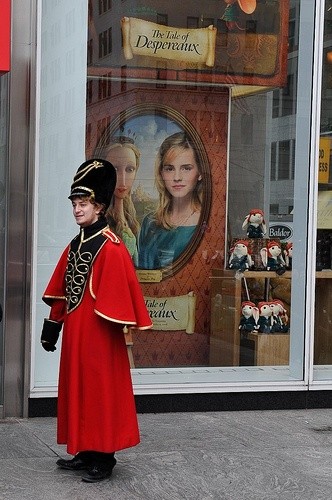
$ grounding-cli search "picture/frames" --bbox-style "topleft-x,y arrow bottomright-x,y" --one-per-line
94,102 -> 212,283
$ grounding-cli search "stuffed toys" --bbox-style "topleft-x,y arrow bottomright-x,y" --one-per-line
239,299 -> 289,336
228,208 -> 292,275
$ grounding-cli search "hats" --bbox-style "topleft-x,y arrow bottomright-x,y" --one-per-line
68,158 -> 116,211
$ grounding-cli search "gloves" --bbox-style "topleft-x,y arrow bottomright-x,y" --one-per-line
40,318 -> 62,352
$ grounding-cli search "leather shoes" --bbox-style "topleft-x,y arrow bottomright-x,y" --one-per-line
56,452 -> 96,469
82,466 -> 113,483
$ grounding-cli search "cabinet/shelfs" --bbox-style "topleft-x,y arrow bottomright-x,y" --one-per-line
208,268 -> 332,367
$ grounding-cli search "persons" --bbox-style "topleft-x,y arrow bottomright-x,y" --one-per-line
39,158 -> 155,484
132,131 -> 207,270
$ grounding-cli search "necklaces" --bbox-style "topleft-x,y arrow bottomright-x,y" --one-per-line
161,202 -> 198,229
